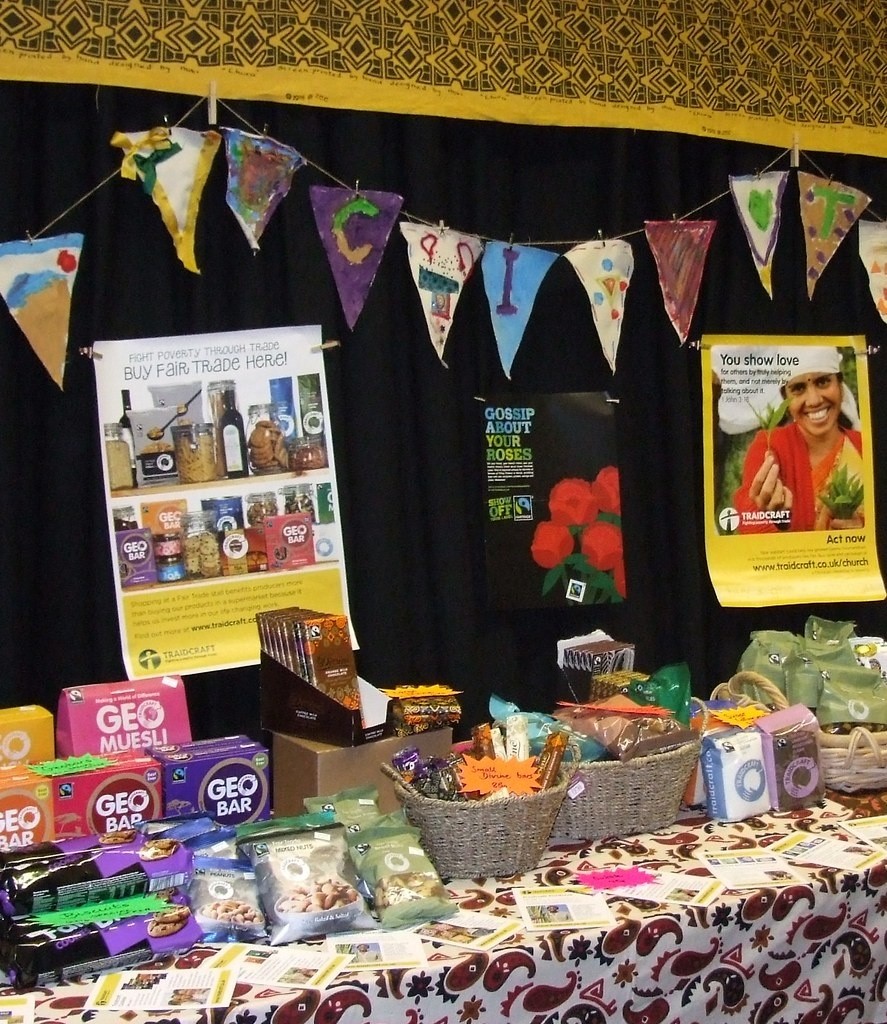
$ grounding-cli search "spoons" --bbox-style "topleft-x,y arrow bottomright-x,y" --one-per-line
147,414 -> 177,440
178,390 -> 201,416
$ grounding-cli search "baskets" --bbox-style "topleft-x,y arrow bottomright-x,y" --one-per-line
381,740 -> 581,880
543,696 -> 708,848
718,670 -> 887,792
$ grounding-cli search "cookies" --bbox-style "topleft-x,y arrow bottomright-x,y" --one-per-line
182,533 -> 220,578
248,420 -> 289,469
98,829 -> 191,938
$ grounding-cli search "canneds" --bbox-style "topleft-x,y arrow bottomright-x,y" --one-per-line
152,532 -> 188,584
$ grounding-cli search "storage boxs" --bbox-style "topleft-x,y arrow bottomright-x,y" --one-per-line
271,727 -> 454,815
0,749 -> 163,850
260,650 -> 393,748
144,734 -> 261,817
0,705 -> 55,767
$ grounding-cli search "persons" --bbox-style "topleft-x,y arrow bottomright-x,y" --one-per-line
545,905 -> 571,922
168,988 -> 211,1006
351,944 -> 379,963
430,921 -> 478,938
732,347 -> 865,535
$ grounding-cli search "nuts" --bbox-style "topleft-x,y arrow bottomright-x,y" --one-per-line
200,878 -> 360,925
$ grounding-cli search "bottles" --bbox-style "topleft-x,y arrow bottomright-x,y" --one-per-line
105,423 -> 133,491
277,482 -> 315,524
113,506 -> 138,533
220,388 -> 248,478
181,510 -> 221,581
247,404 -> 289,477
288,436 -> 327,471
207,379 -> 236,478
247,491 -> 278,531
120,389 -> 138,487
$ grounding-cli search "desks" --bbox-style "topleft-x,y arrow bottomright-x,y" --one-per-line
0,786 -> 887,1024
